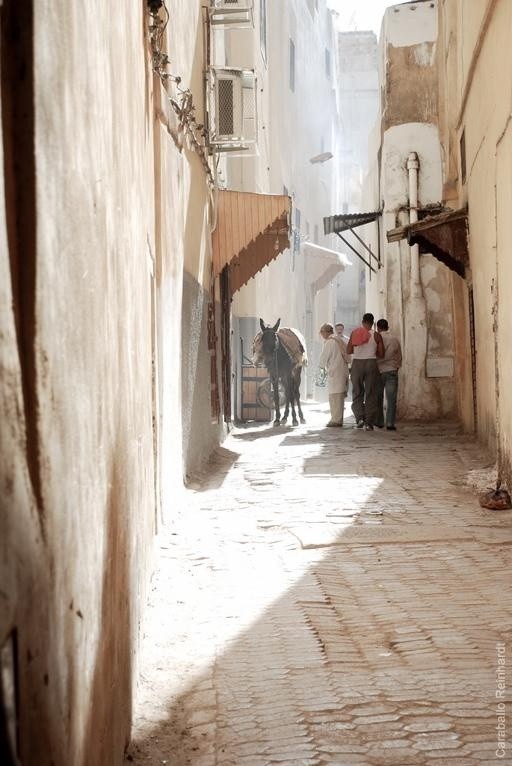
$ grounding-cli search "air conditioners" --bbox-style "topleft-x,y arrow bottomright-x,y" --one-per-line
214,65 -> 259,150
211,1 -> 256,31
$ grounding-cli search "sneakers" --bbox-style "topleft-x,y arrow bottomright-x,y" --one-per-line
326,421 -> 342,427
357,419 -> 396,430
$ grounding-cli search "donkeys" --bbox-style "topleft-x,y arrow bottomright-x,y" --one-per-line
256,317 -> 306,427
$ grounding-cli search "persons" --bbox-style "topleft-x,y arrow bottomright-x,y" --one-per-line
374,319 -> 402,430
335,323 -> 351,397
319,322 -> 348,427
346,313 -> 385,432
